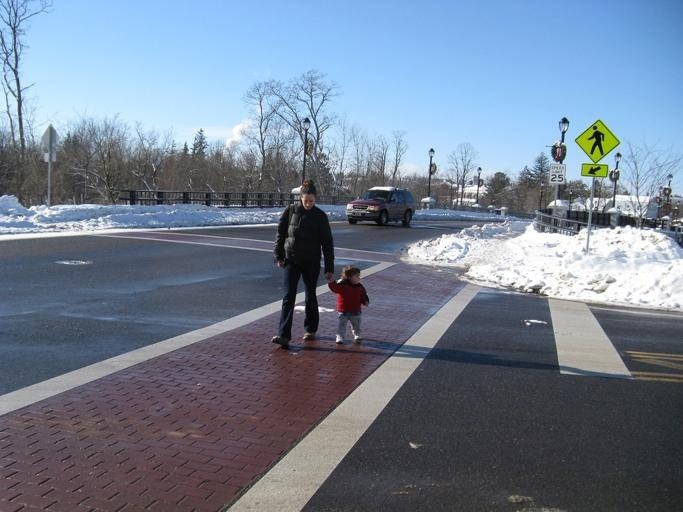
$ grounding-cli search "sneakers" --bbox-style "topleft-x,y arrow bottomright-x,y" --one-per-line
271,336 -> 290,346
352,329 -> 360,340
335,334 -> 345,343
302,332 -> 316,340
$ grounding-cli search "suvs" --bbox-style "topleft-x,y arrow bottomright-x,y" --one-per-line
345,186 -> 415,226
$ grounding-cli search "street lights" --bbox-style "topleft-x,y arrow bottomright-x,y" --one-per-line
609,153 -> 621,208
298,118 -> 311,187
475,167 -> 481,202
427,148 -> 435,198
568,189 -> 572,201
549,116 -> 569,234
538,181 -> 544,201
657,173 -> 681,230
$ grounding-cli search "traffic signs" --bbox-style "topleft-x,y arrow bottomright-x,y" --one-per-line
549,165 -> 565,185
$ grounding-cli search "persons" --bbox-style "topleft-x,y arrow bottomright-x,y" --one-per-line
327,265 -> 369,344
271,179 -> 334,344
